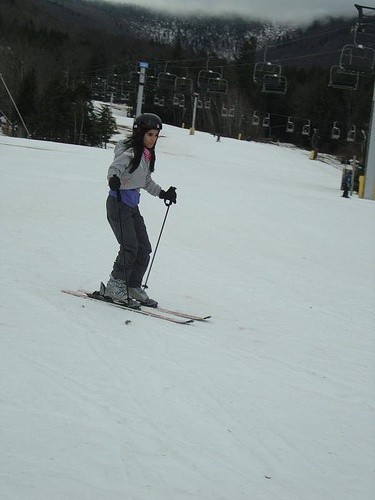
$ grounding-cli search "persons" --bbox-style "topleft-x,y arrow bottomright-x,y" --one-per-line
105,113 -> 177,305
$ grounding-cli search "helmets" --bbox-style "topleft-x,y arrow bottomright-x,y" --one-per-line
133,113 -> 164,133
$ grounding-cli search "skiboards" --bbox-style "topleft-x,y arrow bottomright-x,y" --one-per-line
61,289 -> 194,324
78,288 -> 211,321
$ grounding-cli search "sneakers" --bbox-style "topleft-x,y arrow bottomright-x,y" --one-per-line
126,284 -> 148,303
103,273 -> 132,305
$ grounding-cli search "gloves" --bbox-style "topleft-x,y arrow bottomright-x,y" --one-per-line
109,177 -> 122,192
159,186 -> 177,202
